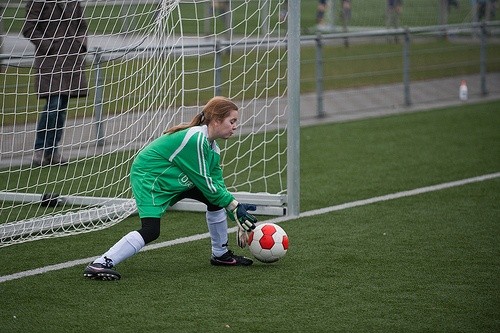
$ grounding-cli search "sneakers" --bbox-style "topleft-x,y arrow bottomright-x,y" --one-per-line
82,261 -> 121,281
211,250 -> 253,266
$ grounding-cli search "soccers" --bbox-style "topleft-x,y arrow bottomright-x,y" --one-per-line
249,221 -> 290,262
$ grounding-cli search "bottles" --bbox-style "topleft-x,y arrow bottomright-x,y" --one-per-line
460,79 -> 468,101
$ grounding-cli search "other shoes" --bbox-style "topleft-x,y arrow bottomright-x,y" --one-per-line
33,148 -> 68,165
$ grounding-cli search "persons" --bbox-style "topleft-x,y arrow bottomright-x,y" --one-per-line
83,95 -> 258,281
209,0 -> 499,41
22,0 -> 89,167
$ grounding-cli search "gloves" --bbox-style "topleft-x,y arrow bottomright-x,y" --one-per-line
226,199 -> 257,233
236,226 -> 249,249
